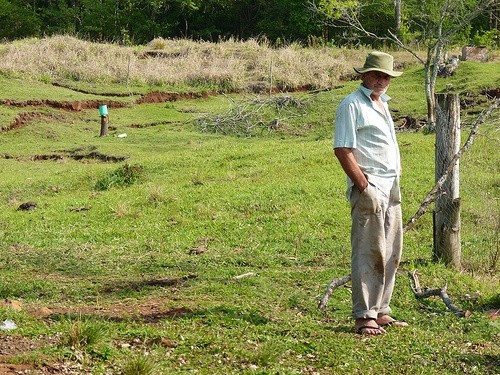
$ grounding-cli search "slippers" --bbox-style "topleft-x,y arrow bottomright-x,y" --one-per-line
355,324 -> 386,336
378,320 -> 409,326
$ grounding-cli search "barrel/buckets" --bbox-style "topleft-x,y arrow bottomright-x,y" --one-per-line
99,105 -> 109,115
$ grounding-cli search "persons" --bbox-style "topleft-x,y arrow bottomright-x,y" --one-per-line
333,51 -> 409,337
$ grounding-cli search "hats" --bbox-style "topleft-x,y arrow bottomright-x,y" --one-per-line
353,52 -> 403,77
